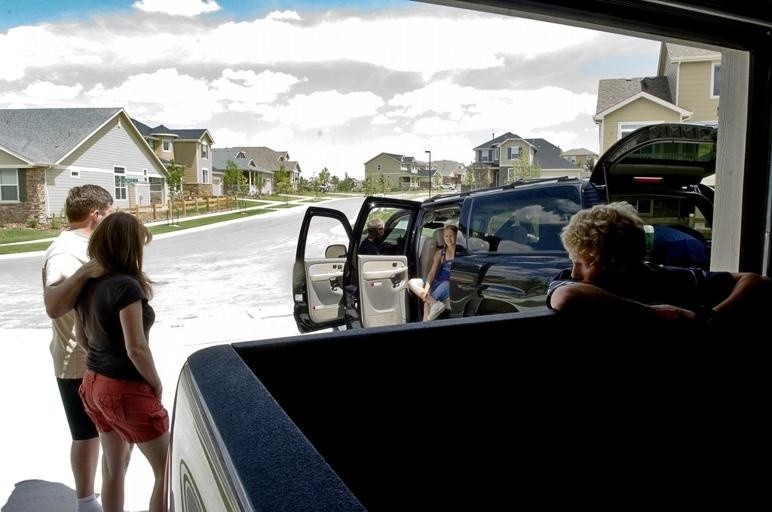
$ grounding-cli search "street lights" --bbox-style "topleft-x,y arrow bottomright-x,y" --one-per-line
422,149 -> 432,199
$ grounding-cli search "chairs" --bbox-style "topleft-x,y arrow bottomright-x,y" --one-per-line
503,226 -> 529,245
420,229 -> 445,287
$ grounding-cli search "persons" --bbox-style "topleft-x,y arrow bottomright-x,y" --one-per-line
545,199 -> 764,325
360,217 -> 386,256
407,224 -> 457,321
74,213 -> 170,511
43,184 -> 109,511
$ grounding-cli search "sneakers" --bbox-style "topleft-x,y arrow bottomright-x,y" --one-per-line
427,300 -> 447,320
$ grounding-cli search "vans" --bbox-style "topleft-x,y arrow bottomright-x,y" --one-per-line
290,122 -> 719,335
